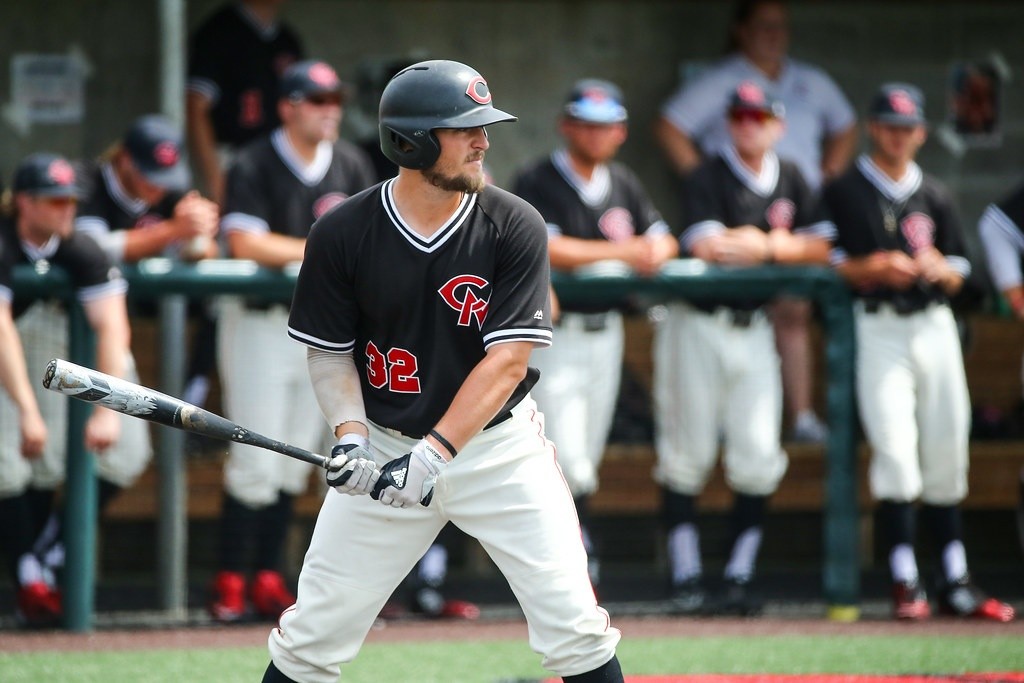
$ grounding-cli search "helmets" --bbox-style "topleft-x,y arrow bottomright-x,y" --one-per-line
377,58 -> 518,170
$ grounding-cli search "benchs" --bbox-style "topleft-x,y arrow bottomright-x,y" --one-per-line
104,312 -> 1024,574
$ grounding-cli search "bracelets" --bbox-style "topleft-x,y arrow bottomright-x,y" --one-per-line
430,430 -> 457,458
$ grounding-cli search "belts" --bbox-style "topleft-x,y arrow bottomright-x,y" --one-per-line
864,299 -> 928,316
398,410 -> 515,440
552,312 -> 607,332
696,302 -> 751,325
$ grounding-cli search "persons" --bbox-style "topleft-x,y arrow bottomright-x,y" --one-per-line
190,0 -> 310,205
977,181 -> 1024,315
212,59 -> 478,622
651,82 -> 837,618
504,81 -> 678,500
822,85 -> 1015,625
261,60 -> 625,683
1,119 -> 218,626
652,0 -> 859,441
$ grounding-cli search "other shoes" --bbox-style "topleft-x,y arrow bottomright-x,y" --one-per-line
19,548 -> 63,624
721,573 -> 760,617
937,576 -> 1015,623
890,586 -> 933,621
210,571 -> 246,625
252,567 -> 291,613
421,568 -> 448,619
674,570 -> 710,617
793,408 -> 829,440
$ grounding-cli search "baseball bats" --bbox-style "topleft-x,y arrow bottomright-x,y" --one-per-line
39,357 -> 437,513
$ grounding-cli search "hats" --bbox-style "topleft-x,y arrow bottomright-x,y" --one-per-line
12,152 -> 81,201
561,76 -> 630,125
280,58 -> 359,100
864,81 -> 929,126
122,110 -> 195,192
729,83 -> 783,119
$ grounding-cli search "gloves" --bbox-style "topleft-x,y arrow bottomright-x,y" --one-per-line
325,434 -> 379,498
372,438 -> 449,509
